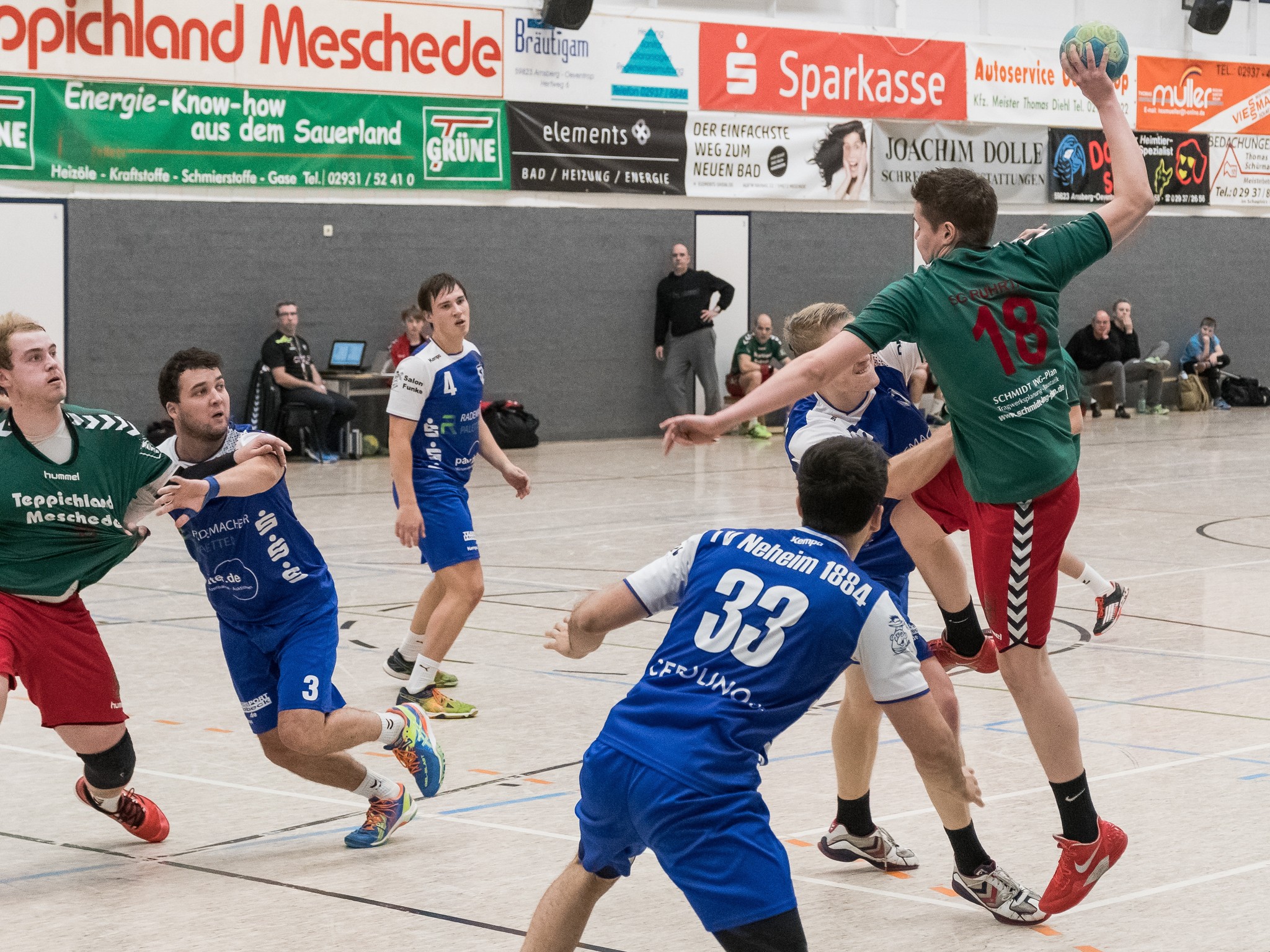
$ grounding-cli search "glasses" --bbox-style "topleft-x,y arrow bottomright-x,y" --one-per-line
276,311 -> 298,319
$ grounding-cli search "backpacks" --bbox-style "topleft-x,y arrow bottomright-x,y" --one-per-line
1176,373 -> 1210,413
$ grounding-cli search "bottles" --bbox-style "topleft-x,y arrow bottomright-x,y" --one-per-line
1137,383 -> 1146,413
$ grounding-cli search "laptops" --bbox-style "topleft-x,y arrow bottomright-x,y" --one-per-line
318,340 -> 367,374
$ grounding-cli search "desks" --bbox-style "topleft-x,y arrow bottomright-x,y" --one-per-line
317,371 -> 397,459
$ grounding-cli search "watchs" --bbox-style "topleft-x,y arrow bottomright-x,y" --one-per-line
714,306 -> 721,314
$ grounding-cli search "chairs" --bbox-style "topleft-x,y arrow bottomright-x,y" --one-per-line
262,364 -> 324,463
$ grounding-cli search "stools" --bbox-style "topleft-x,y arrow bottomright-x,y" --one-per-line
722,396 -> 766,436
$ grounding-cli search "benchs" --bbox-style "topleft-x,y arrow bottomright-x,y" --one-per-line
1100,371 -> 1226,410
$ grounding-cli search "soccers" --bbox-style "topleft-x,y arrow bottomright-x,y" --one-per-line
1059,21 -> 1130,84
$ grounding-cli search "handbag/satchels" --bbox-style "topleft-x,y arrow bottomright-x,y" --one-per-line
1244,378 -> 1270,406
1221,375 -> 1249,407
481,401 -> 539,450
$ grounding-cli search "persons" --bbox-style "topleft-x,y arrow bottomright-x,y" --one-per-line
660,43 -> 1153,913
1,310 -> 293,841
380,273 -> 529,719
389,305 -> 428,369
909,360 -> 947,426
783,300 -> 1051,925
725,313 -> 792,440
1112,297 -> 1231,415
653,243 -> 735,441
515,437 -> 983,952
805,119 -> 868,201
261,300 -> 357,464
1063,310 -> 1131,418
122,348 -> 445,847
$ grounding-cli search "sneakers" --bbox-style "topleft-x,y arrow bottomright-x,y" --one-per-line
1145,404 -> 1170,416
302,441 -> 322,464
381,703 -> 447,798
74,778 -> 169,844
383,648 -> 458,690
342,784 -> 418,850
1090,401 -> 1102,418
1195,361 -> 1211,373
1038,813 -> 1129,918
1092,579 -> 1130,637
1115,406 -> 1132,419
746,422 -> 773,440
1143,357 -> 1171,372
926,411 -> 948,426
320,447 -> 337,464
922,628 -> 1001,676
1212,396 -> 1232,410
394,681 -> 479,721
951,858 -> 1052,924
816,818 -> 919,875
